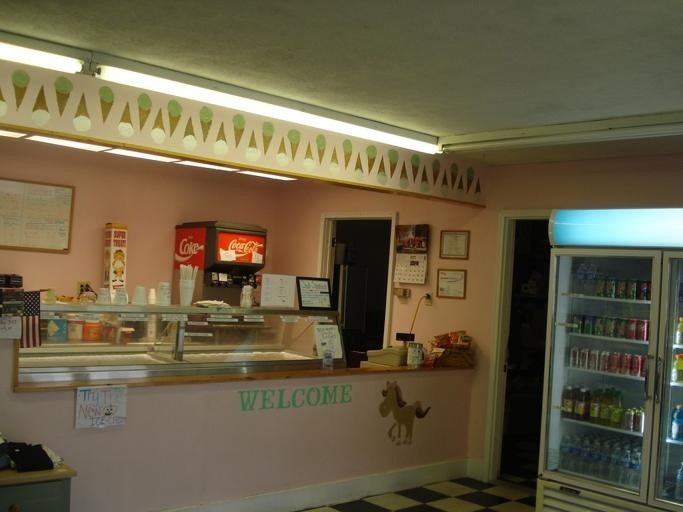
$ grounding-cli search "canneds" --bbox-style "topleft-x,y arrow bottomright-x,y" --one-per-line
624,404 -> 645,434
570,279 -> 651,377
407,343 -> 422,369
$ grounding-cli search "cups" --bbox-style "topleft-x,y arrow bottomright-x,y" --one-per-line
156,280 -> 171,307
406,340 -> 428,371
95,285 -> 156,306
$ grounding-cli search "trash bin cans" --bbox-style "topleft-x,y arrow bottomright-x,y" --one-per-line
1,442 -> 77,511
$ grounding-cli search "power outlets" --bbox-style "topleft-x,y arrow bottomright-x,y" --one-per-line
424,291 -> 432,308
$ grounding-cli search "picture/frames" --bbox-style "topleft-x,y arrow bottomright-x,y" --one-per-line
436,229 -> 471,299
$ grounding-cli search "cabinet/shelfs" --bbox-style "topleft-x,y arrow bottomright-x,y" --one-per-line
0,457 -> 76,512
17,308 -> 345,382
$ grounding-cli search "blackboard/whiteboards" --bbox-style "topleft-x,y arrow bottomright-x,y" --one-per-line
0,178 -> 75,254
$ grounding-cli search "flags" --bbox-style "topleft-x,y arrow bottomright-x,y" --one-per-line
18,290 -> 44,349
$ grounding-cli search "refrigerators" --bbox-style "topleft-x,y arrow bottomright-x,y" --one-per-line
534,244 -> 683,504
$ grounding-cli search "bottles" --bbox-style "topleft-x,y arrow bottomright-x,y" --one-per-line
572,261 -> 599,297
559,383 -> 624,430
559,431 -> 643,491
670,315 -> 683,500
